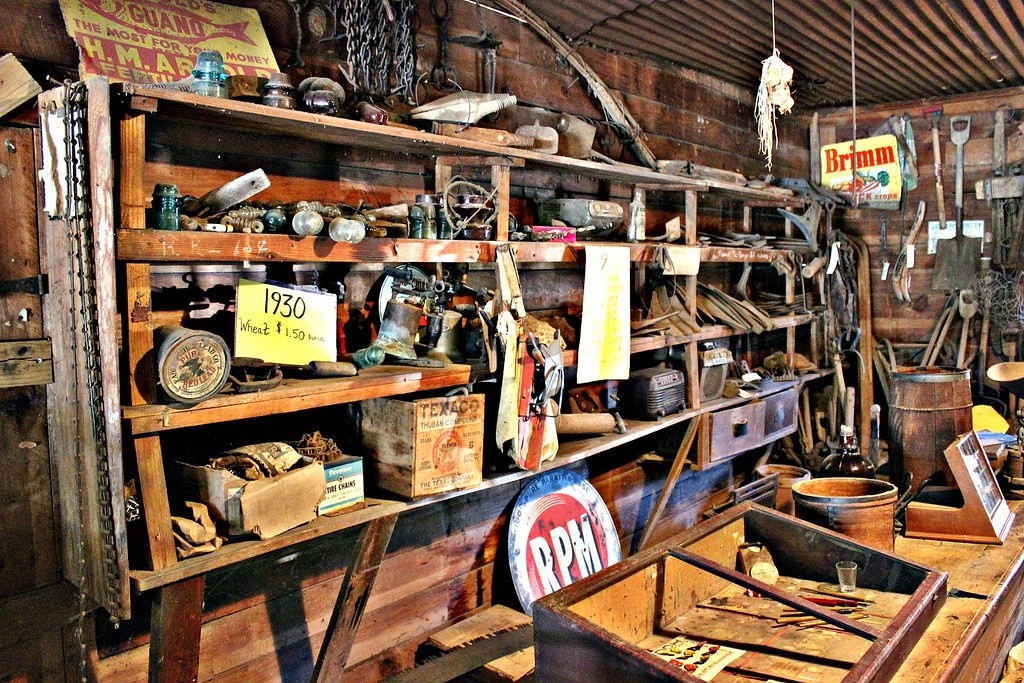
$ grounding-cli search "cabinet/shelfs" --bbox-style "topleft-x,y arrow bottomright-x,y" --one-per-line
38,75 -> 837,683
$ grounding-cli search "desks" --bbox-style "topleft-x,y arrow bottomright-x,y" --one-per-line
890,501 -> 1024,683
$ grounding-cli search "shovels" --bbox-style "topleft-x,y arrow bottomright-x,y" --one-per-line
930,114 -> 983,291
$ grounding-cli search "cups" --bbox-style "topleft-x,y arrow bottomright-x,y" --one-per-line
836,561 -> 858,593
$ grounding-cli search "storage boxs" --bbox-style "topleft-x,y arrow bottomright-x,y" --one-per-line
760,387 -> 797,438
535,501 -> 951,683
173,455 -> 364,537
356,394 -> 487,498
707,400 -> 767,460
529,225 -> 577,243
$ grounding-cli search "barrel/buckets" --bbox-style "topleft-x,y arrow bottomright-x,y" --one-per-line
755,463 -> 811,521
792,477 -> 898,564
888,364 -> 973,504
